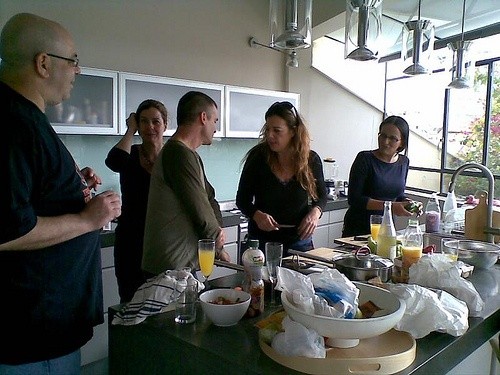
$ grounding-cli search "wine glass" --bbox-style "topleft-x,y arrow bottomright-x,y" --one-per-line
198,239 -> 215,289
264,242 -> 283,308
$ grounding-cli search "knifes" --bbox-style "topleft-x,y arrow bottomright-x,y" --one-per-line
275,224 -> 293,228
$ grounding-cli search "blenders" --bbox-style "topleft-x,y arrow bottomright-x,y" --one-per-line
322,157 -> 338,200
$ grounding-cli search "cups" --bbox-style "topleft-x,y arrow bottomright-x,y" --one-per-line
169,278 -> 198,324
441,238 -> 459,262
370,214 -> 383,244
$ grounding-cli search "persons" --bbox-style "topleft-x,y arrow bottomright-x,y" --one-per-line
139,90 -> 230,276
341,115 -> 424,239
235,101 -> 328,263
0,13 -> 122,375
105,99 -> 168,304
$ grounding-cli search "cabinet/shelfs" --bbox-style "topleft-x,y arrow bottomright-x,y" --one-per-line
80,206 -> 348,369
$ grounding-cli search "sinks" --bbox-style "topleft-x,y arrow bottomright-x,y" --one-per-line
397,233 -> 446,253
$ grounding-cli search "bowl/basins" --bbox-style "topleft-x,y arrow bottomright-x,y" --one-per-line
199,289 -> 251,326
281,279 -> 406,349
444,240 -> 500,269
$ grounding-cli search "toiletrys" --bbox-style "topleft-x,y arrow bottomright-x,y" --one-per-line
426,193 -> 440,232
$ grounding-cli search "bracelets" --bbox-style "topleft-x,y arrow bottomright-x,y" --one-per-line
314,206 -> 322,219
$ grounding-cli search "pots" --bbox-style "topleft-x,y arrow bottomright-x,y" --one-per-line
334,247 -> 393,283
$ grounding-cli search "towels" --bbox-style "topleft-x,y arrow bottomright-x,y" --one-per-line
112,268 -> 205,325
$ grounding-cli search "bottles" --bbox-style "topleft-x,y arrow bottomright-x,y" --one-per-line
402,219 -> 423,283
442,192 -> 458,222
241,240 -> 265,316
426,190 -> 440,235
377,200 -> 397,262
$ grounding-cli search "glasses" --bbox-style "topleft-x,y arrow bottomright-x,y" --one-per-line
270,101 -> 297,118
47,53 -> 79,67
378,133 -> 402,142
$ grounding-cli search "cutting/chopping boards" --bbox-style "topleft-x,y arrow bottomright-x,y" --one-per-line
283,248 -> 349,272
333,233 -> 371,250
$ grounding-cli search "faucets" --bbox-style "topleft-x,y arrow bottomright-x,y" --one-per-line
448,164 -> 500,243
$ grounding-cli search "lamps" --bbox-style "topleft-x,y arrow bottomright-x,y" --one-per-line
345,0 -> 383,62
267,0 -> 313,51
402,0 -> 434,76
446,0 -> 474,90
249,36 -> 300,68
